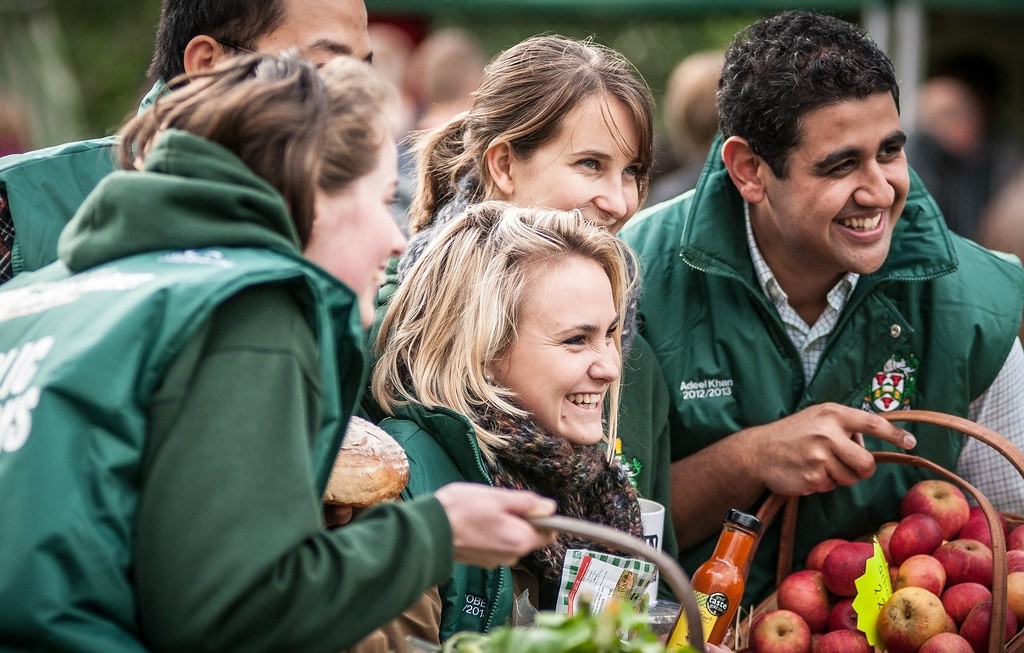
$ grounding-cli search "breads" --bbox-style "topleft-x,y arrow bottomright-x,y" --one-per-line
317,415 -> 411,507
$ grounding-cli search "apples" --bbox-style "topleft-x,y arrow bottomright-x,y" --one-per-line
749,481 -> 1024,653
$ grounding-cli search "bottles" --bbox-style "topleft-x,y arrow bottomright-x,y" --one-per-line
661,508 -> 765,652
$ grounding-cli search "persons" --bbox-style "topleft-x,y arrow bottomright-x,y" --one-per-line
367,22 -> 483,236
617,13 -> 1024,617
364,37 -> 680,606
645,55 -> 726,208
0,0 -> 373,282
357,201 -> 643,653
0,47 -> 556,653
907,77 -> 1005,248
